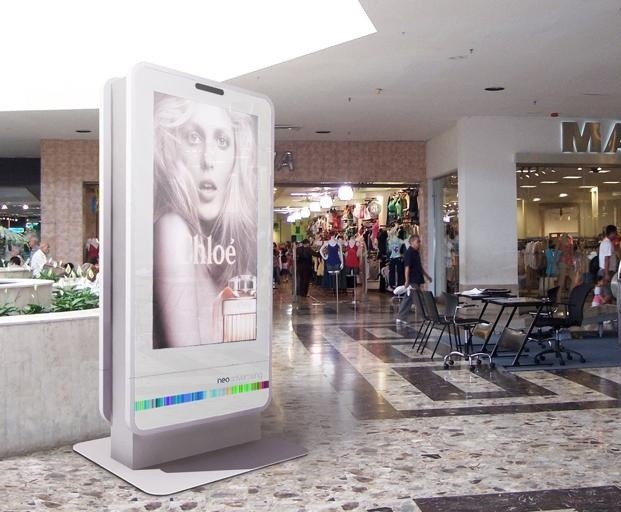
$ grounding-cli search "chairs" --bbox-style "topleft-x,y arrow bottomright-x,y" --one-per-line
408,269 -> 594,373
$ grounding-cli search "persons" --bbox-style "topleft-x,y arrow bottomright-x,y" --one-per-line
387,229 -> 404,286
153,95 -> 256,347
387,190 -> 418,218
396,235 -> 433,324
345,236 -> 360,271
7,237 -> 99,286
319,234 -> 344,271
598,224 -> 619,301
592,276 -> 609,306
273,238 -> 321,296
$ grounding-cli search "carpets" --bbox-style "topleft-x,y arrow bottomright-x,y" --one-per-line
432,328 -> 621,375
